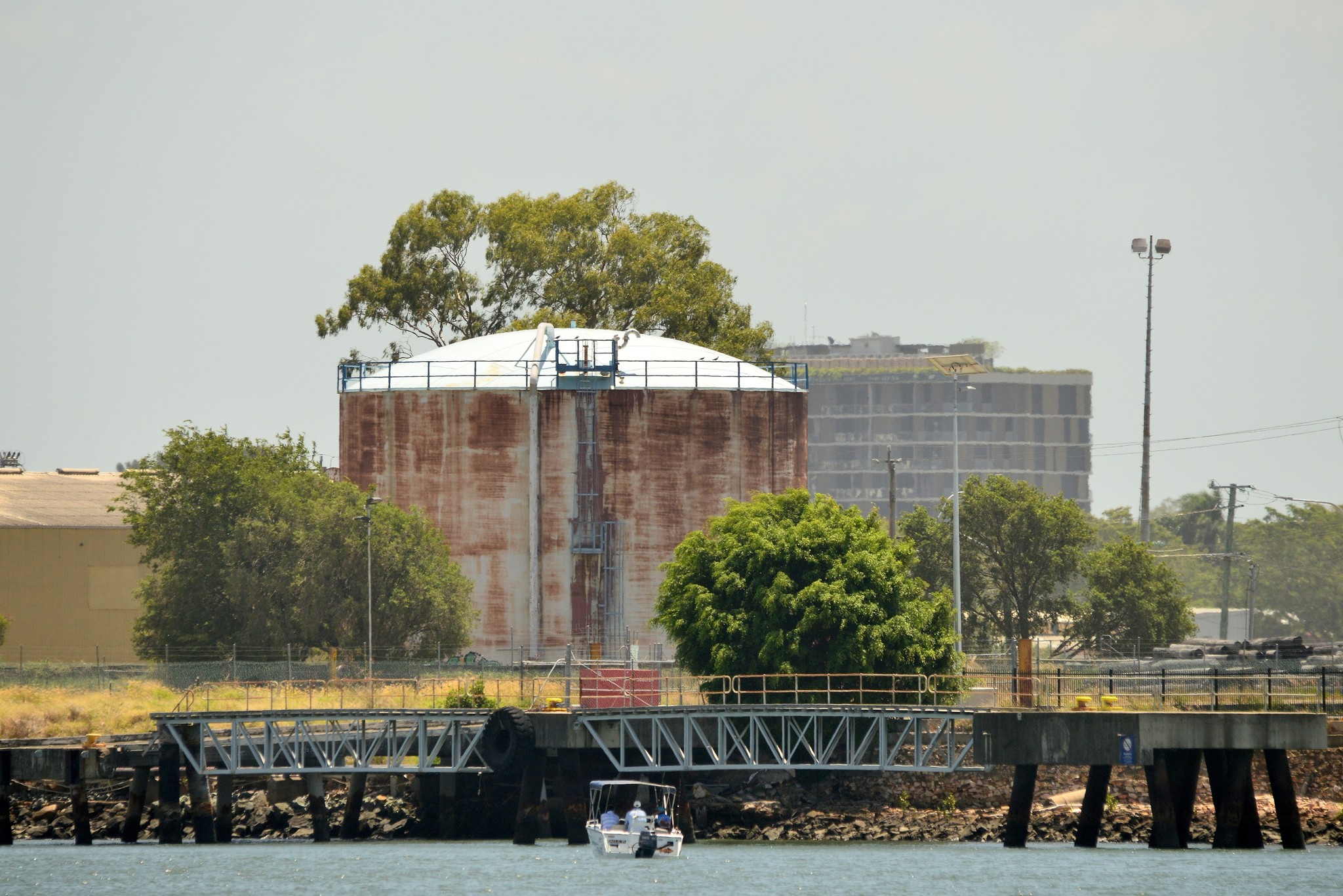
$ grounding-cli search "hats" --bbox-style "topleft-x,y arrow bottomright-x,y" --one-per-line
657,807 -> 664,812
633,801 -> 641,808
607,805 -> 614,810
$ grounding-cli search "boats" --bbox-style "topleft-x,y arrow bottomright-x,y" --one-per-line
585,780 -> 683,859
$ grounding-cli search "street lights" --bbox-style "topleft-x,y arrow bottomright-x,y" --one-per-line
1131,235 -> 1172,546
925,353 -> 992,676
352,497 -> 383,688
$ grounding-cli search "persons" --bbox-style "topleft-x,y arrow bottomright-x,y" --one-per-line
625,801 -> 646,833
600,806 -> 620,831
656,807 -> 670,828
